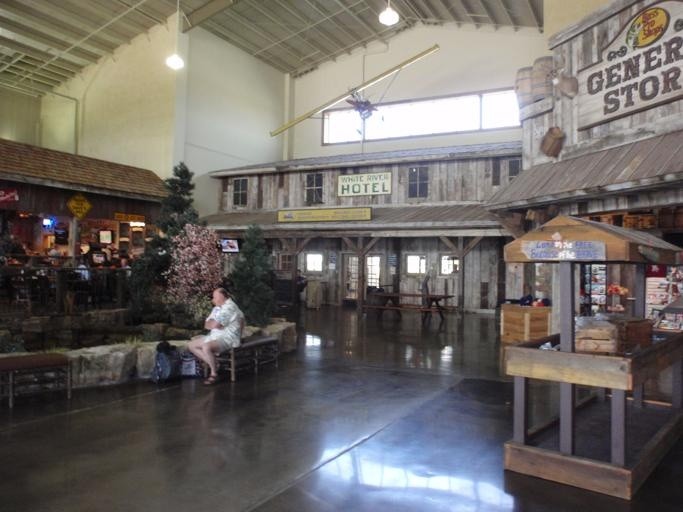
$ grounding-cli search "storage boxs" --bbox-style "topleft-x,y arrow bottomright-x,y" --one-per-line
574,318 -> 654,356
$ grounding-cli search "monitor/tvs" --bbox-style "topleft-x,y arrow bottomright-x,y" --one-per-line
100,229 -> 113,245
218,238 -> 241,254
91,252 -> 107,265
54,229 -> 69,245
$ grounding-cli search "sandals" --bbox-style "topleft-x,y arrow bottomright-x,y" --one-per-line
202,373 -> 219,385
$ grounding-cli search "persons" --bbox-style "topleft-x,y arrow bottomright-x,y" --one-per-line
0,241 -> 132,281
451,264 -> 458,273
296,270 -> 308,306
188,289 -> 246,385
520,283 -> 533,306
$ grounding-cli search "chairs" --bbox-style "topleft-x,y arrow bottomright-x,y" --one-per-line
1,263 -> 135,317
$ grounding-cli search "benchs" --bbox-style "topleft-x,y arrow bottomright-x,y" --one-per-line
364,303 -> 458,322
0,353 -> 73,409
204,334 -> 279,382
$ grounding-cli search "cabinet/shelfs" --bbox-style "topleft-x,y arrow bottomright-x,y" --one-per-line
501,210 -> 683,500
501,303 -> 552,344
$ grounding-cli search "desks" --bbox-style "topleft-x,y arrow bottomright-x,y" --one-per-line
369,291 -> 454,319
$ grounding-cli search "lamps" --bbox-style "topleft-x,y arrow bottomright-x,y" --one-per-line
166,0 -> 184,71
378,0 -> 399,26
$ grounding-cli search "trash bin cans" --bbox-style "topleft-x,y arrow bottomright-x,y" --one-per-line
306,277 -> 321,309
